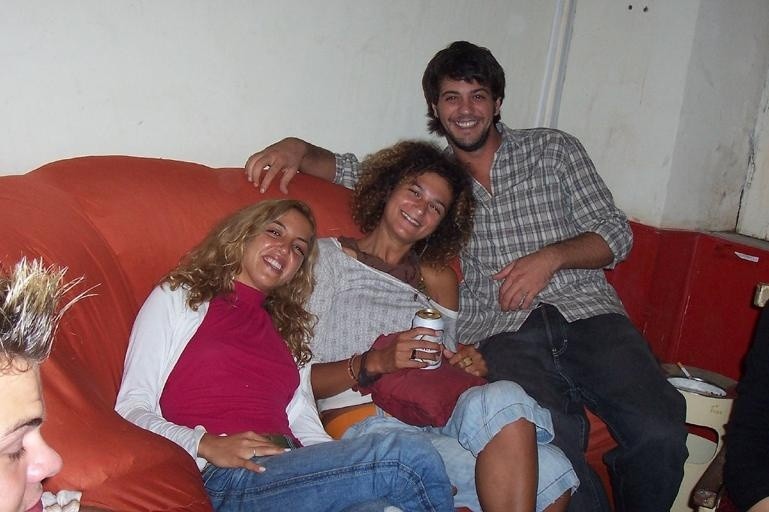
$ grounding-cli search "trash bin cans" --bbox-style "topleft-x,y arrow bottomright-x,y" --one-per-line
655,363 -> 738,512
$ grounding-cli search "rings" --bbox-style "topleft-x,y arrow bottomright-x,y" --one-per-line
411,349 -> 417,360
252,446 -> 257,458
462,355 -> 474,367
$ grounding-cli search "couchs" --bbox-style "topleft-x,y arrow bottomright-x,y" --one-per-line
0,154 -> 618,510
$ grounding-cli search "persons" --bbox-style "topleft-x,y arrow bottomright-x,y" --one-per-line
0,254 -> 100,512
304,138 -> 582,512
113,198 -> 458,512
720,300 -> 769,511
244,38 -> 692,511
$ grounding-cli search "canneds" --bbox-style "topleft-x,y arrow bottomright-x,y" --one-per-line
411,310 -> 444,370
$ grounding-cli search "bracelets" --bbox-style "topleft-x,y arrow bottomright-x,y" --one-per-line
357,350 -> 384,384
347,352 -> 361,386
